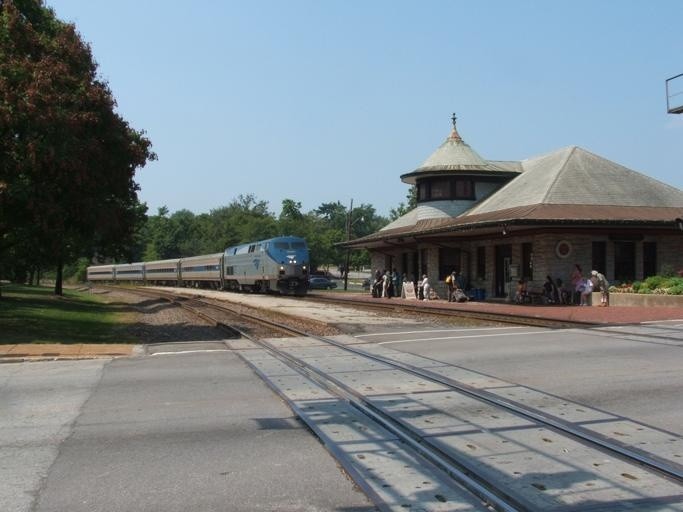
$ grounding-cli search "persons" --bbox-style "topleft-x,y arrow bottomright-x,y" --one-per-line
372,268 -> 431,301
515,277 -> 527,304
543,264 -> 609,307
447,271 -> 457,302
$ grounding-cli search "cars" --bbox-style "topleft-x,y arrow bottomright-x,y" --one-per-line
306,277 -> 337,291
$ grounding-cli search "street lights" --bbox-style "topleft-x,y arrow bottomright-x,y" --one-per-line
344,215 -> 366,290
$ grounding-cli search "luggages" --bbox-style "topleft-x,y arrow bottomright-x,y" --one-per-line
453,288 -> 467,302
372,283 -> 379,298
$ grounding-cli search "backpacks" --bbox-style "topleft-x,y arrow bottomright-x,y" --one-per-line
445,275 -> 452,285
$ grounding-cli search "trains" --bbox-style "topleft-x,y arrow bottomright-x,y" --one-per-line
87,234 -> 311,300
